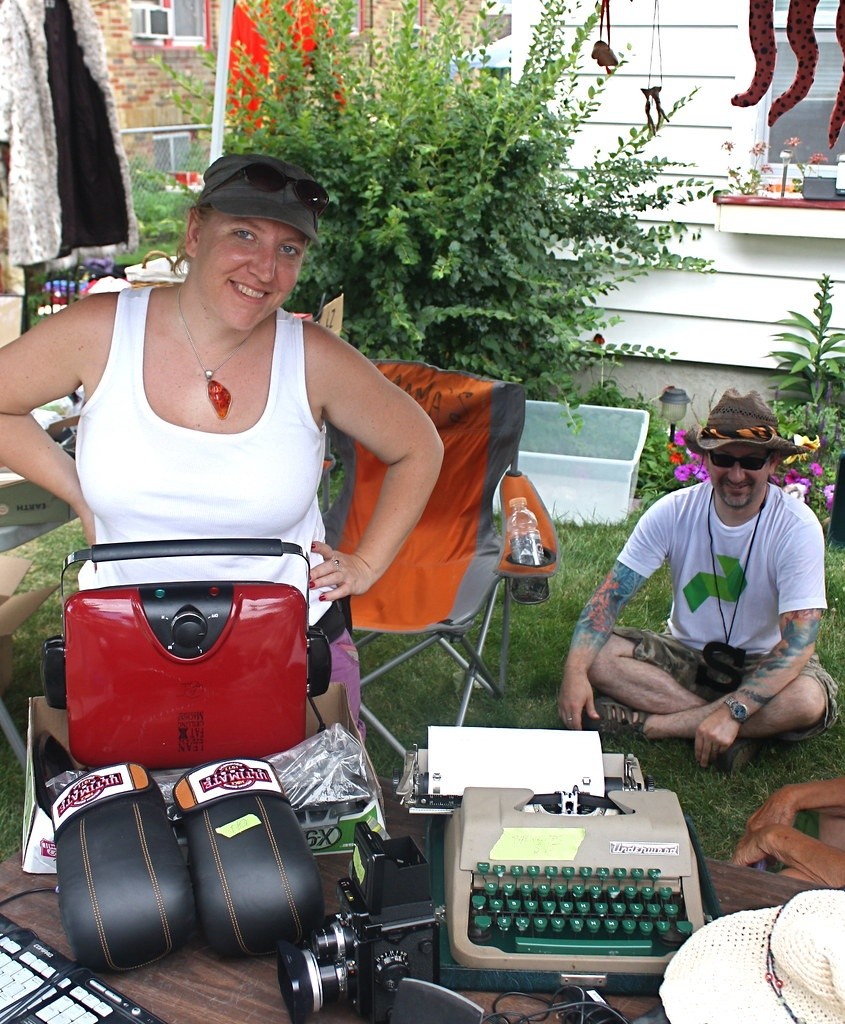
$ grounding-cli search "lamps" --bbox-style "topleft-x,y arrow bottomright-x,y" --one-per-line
658,387 -> 692,441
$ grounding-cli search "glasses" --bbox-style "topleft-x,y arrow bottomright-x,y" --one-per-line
709,449 -> 775,470
201,161 -> 330,219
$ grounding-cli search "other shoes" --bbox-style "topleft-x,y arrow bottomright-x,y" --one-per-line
828,537 -> 844,552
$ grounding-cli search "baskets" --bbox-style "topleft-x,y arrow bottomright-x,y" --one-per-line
125,250 -> 190,288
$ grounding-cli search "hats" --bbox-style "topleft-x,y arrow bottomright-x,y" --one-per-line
658,886 -> 845,1024
195,153 -> 331,250
695,386 -> 810,456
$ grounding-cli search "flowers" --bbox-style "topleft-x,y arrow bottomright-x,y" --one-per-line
722,141 -> 777,197
784,137 -> 829,194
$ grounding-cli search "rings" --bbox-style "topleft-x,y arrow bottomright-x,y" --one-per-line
331,558 -> 340,572
567,718 -> 572,720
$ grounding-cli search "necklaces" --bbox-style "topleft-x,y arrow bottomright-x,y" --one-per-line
177,286 -> 247,419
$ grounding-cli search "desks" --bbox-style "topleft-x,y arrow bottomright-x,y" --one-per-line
1,785 -> 844,1024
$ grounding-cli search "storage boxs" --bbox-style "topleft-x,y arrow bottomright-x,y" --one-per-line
0,555 -> 60,698
492,400 -> 650,527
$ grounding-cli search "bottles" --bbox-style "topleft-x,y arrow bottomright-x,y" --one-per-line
506,497 -> 544,567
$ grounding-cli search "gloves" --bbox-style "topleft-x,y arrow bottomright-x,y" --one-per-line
168,758 -> 324,962
51,764 -> 198,974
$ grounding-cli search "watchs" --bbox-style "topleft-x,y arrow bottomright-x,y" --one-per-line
724,696 -> 750,724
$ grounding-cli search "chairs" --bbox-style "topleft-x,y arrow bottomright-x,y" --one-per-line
317,358 -> 526,785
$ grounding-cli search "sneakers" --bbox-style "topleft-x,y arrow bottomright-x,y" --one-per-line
718,737 -> 765,778
582,695 -> 650,747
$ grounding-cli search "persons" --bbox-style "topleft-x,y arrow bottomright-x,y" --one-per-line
556,388 -> 838,773
731,775 -> 845,888
1,154 -> 445,742
